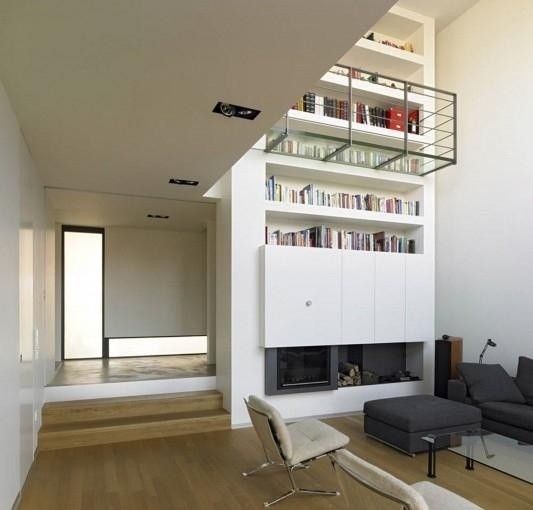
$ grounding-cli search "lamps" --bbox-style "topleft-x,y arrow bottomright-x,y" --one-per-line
478,338 -> 496,367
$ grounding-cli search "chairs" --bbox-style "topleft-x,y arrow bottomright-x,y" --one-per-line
239,395 -> 351,508
326,448 -> 486,510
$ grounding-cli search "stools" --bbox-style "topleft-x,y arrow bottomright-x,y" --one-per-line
362,393 -> 481,456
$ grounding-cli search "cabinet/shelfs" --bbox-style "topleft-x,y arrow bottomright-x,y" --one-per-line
266,12 -> 425,253
259,245 -> 437,348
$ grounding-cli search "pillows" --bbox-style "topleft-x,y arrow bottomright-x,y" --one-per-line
455,357 -> 532,404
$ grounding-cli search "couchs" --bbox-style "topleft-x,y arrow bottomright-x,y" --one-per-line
445,376 -> 533,451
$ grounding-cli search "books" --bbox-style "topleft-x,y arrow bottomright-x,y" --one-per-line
265,30 -> 422,253
